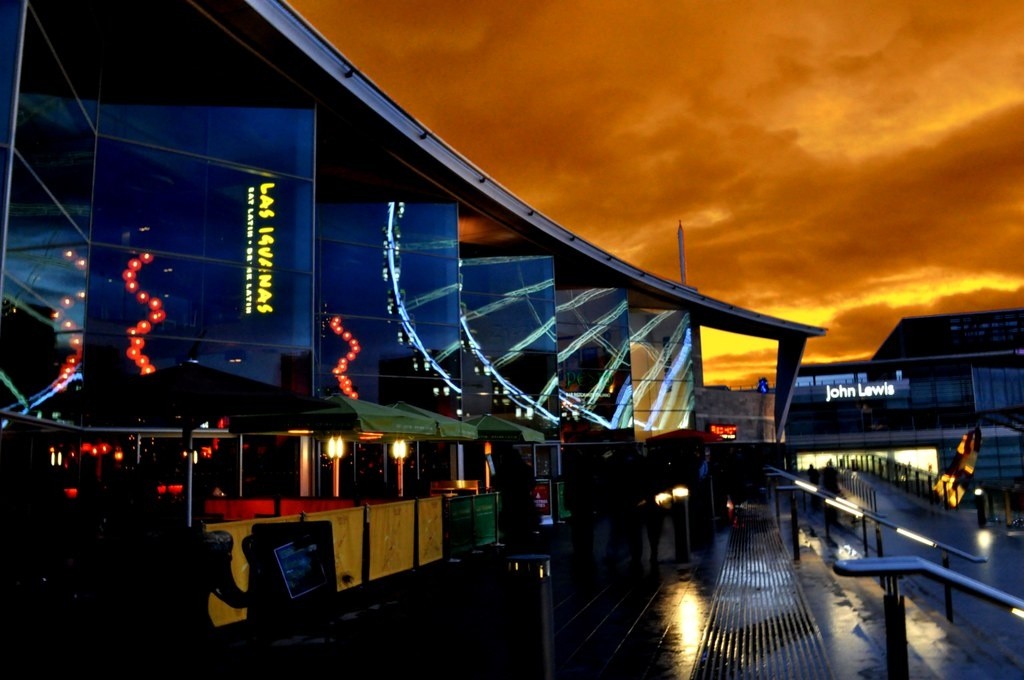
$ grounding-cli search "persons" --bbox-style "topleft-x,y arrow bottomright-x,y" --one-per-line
496,475 -> 541,575
563,472 -> 646,579
807,464 -> 820,486
640,470 -> 673,568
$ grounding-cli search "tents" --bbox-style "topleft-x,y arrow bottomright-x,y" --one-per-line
75,363 -> 548,525
